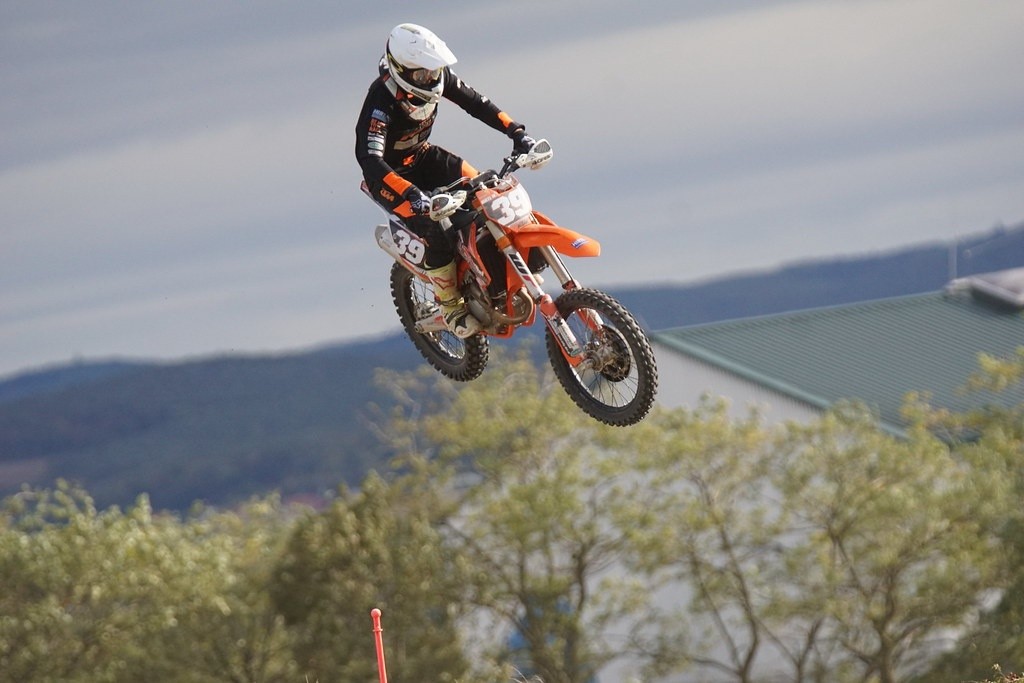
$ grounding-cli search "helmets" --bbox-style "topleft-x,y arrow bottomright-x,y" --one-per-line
386,22 -> 457,104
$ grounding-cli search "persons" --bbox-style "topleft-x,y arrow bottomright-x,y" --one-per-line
355,23 -> 536,339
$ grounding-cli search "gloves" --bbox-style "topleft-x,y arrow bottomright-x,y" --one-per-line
513,130 -> 536,153
407,187 -> 431,216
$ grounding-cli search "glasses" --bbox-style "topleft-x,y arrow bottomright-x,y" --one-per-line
408,67 -> 442,85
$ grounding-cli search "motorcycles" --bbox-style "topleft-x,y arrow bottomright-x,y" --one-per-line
358,133 -> 661,427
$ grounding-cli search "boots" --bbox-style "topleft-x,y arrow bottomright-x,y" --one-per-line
424,256 -> 482,339
